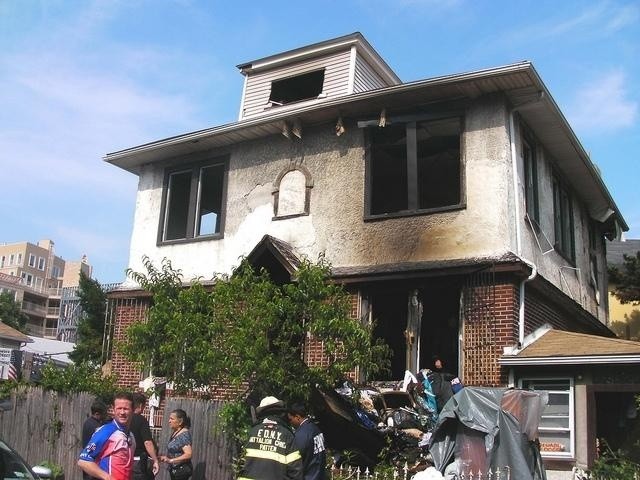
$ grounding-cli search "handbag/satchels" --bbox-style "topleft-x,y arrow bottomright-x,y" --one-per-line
169,460 -> 193,480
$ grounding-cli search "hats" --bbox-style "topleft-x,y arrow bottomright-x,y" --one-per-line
255,396 -> 284,418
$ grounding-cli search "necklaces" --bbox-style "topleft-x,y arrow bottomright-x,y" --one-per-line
168,426 -> 183,444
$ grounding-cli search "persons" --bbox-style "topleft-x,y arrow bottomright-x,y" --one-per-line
236,396 -> 326,480
76,391 -> 160,480
424,355 -> 462,412
158,409 -> 193,480
